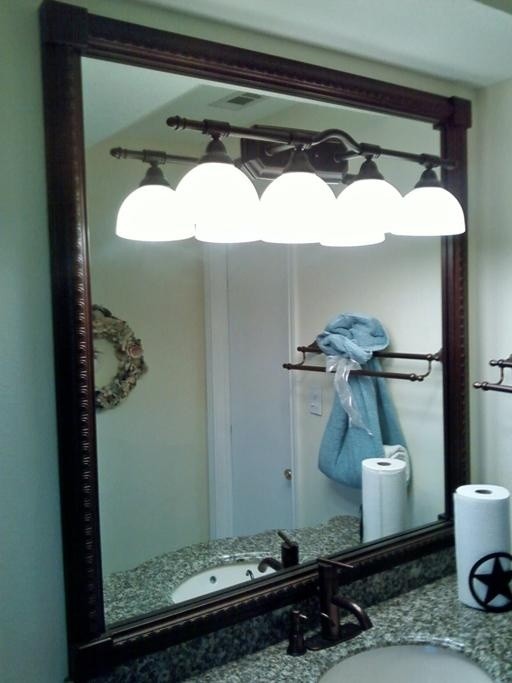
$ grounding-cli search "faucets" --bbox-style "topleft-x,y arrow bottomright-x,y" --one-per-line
245,569 -> 255,581
258,531 -> 299,571
288,608 -> 337,656
317,558 -> 373,639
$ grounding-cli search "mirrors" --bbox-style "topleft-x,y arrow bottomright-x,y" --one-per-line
40,4 -> 472,681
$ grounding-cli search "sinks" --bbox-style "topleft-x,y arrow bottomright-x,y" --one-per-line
170,558 -> 279,605
313,639 -> 499,683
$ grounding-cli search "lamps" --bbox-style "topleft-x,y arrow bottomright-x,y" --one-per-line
165,115 -> 468,238
110,144 -> 385,248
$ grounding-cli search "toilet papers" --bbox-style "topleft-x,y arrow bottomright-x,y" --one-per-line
453,483 -> 510,612
361,457 -> 407,543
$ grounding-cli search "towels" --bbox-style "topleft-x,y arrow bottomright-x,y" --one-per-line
313,313 -> 413,490
